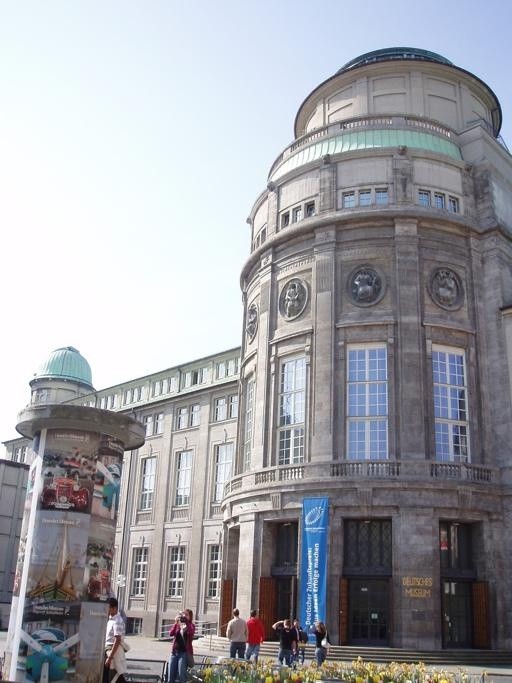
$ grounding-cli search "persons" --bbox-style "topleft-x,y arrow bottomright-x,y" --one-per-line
164,608 -> 196,682
290,618 -> 301,664
309,620 -> 331,666
225,607 -> 249,659
296,627 -> 309,666
245,608 -> 266,667
99,595 -> 128,683
271,618 -> 297,668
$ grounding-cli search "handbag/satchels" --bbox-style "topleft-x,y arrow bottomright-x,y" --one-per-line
187,654 -> 195,668
320,639 -> 331,648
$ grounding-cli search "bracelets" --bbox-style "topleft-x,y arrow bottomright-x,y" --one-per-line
106,655 -> 113,658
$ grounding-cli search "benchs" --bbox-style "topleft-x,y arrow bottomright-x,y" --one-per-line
122,658 -> 168,683
